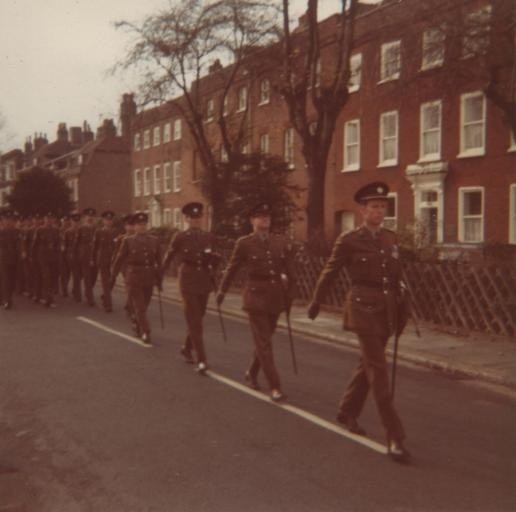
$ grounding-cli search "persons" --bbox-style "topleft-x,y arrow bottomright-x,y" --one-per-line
0,209 -> 161,343
160,202 -> 222,371
308,180 -> 411,462
216,202 -> 289,403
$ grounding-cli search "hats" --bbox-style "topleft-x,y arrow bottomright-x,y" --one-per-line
83,207 -> 96,217
179,201 -> 204,220
101,211 -> 115,220
0,204 -> 81,221
244,202 -> 274,217
121,214 -> 134,225
352,181 -> 390,206
134,212 -> 149,224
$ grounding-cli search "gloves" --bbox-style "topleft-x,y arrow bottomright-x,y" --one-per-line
307,302 -> 319,319
214,292 -> 226,306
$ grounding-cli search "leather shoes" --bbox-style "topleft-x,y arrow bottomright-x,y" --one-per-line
134,324 -> 152,344
244,371 -> 259,390
269,387 -> 287,403
180,344 -> 208,376
336,410 -> 367,437
387,437 -> 411,461
3,287 -> 113,314
